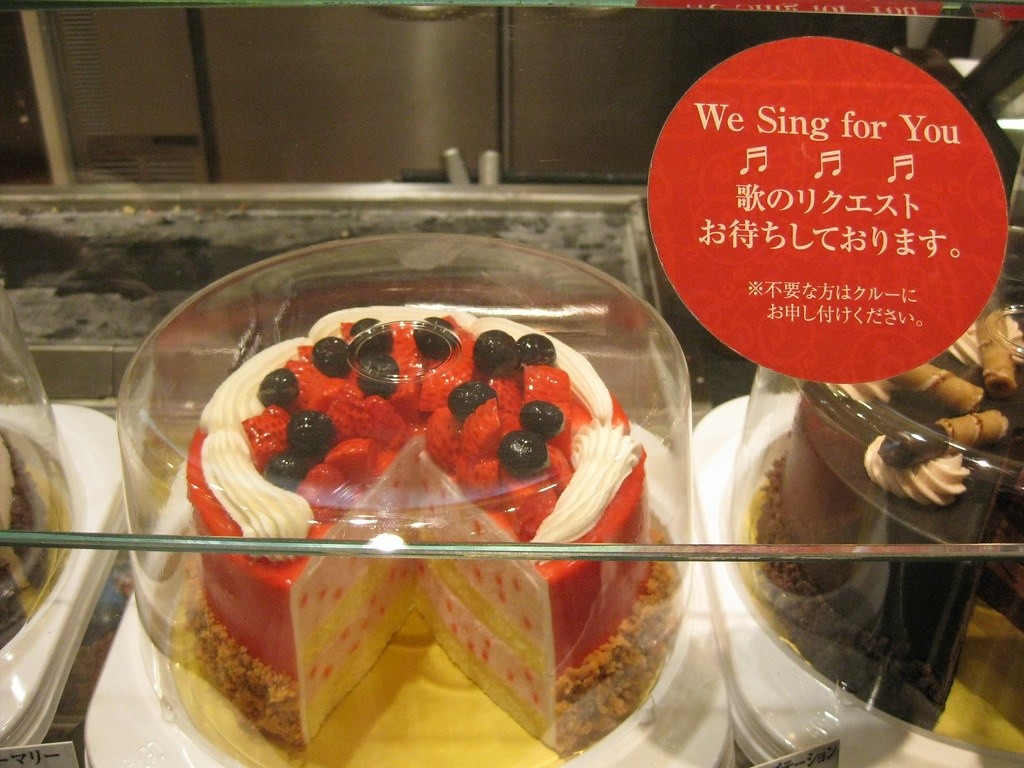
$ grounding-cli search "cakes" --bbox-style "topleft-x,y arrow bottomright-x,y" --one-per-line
745,313 -> 1024,729
180,303 -> 676,758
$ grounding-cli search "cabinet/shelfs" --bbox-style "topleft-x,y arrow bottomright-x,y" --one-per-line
194,7 -> 697,191
